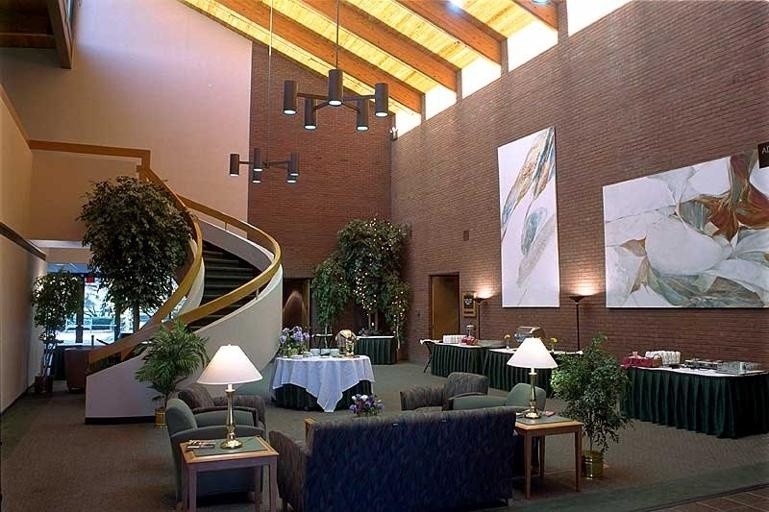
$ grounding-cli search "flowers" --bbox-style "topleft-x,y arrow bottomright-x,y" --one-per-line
350,394 -> 384,417
280,326 -> 310,358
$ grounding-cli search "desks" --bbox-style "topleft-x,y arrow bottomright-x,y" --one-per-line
487,349 -> 582,397
514,411 -> 584,499
354,336 -> 397,364
177,436 -> 280,512
430,340 -> 507,377
618,364 -> 769,439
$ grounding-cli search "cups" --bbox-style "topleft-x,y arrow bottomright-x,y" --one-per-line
717,361 -> 762,375
303,349 -> 340,358
443,335 -> 467,344
645,351 -> 680,365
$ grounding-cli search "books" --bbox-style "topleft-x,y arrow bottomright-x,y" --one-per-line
186,439 -> 216,449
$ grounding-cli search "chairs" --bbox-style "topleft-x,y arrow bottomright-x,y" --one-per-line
165,398 -> 265,505
400,372 -> 489,411
177,382 -> 266,427
453,383 -> 546,413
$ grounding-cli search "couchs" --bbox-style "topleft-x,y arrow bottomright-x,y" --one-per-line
270,407 -> 516,512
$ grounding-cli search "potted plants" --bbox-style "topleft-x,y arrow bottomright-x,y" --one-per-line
549,332 -> 638,480
133,318 -> 211,426
30,267 -> 84,397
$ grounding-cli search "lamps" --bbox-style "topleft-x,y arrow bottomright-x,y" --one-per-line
472,298 -> 487,340
506,338 -> 558,419
568,295 -> 585,351
197,344 -> 264,449
283,1 -> 389,130
229,0 -> 301,184
